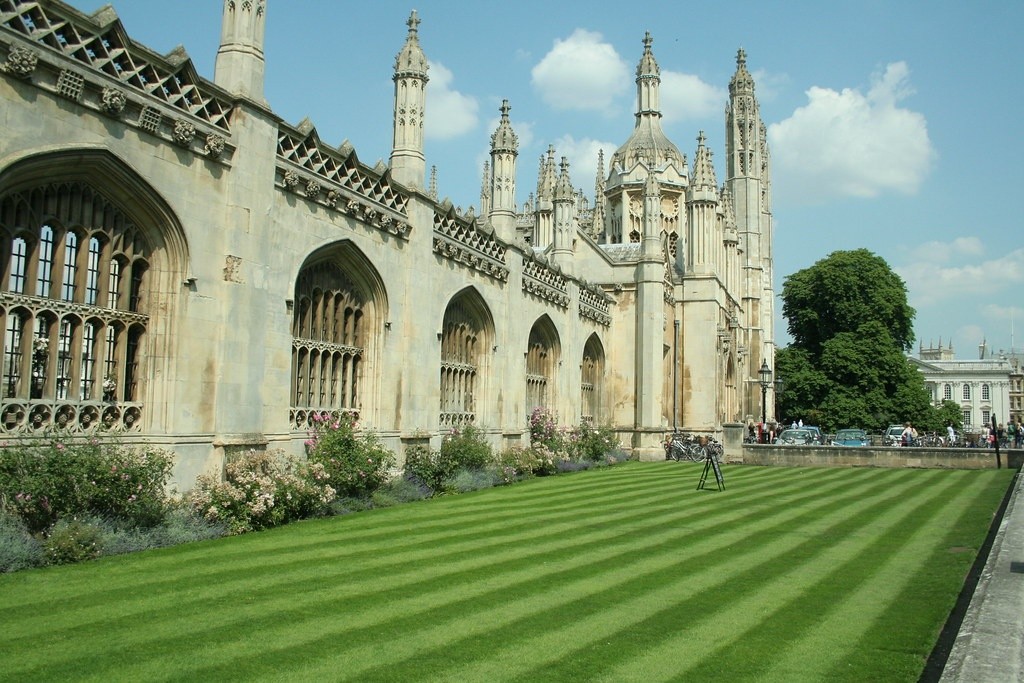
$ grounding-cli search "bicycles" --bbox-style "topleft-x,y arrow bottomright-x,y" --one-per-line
660,432 -> 724,462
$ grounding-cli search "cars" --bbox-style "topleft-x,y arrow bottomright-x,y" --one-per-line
881,425 -> 906,446
798,426 -> 825,446
831,430 -> 871,447
775,429 -> 813,446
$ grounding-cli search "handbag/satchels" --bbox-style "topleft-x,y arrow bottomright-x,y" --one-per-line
904,434 -> 912,442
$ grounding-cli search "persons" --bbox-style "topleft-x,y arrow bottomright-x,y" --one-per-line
791,418 -> 804,429
901,421 -> 918,446
740,420 -> 749,443
980,422 -> 1024,449
748,421 -> 756,444
769,423 -> 775,444
776,424 -> 782,442
946,421 -> 956,447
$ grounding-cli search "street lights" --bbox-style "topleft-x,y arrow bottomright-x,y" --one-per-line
757,357 -> 772,432
